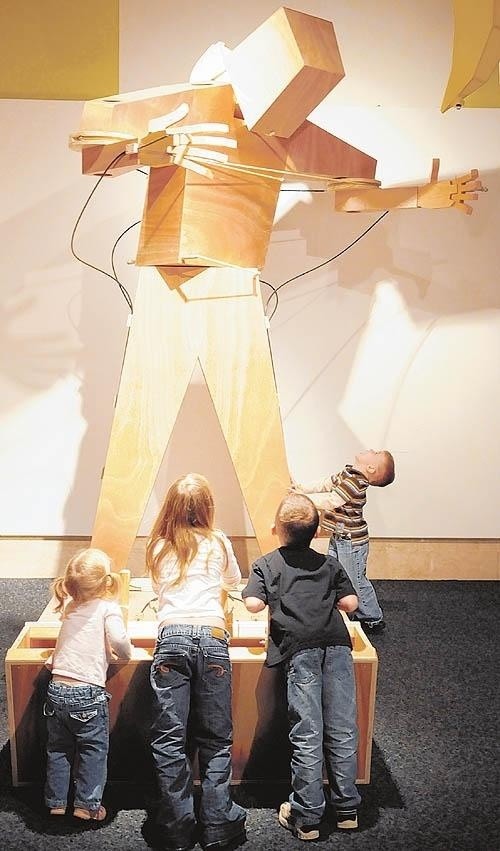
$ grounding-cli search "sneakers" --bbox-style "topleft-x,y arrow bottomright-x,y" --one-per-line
353,614 -> 388,636
278,799 -> 323,841
335,805 -> 360,831
50,804 -> 108,822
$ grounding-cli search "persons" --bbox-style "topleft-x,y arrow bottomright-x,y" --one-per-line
40,548 -> 135,824
144,471 -> 248,850
240,492 -> 363,840
288,447 -> 397,635
